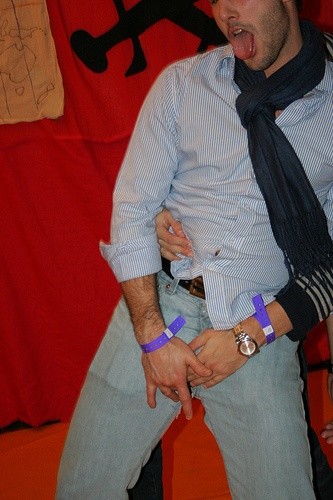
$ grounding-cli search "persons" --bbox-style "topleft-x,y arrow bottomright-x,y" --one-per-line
125,208 -> 332,500
54,0 -> 332,499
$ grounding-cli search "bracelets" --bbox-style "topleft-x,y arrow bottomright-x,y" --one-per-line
251,294 -> 276,346
140,315 -> 186,353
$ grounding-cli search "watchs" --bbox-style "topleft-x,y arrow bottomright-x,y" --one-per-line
232,323 -> 260,359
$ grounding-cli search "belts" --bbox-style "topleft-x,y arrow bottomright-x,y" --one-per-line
161,258 -> 205,300
